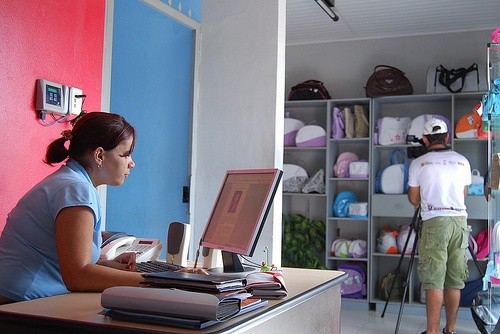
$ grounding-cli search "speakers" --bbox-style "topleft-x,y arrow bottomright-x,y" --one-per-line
166,221 -> 191,267
202,246 -> 224,273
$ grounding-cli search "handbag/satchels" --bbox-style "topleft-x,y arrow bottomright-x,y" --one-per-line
335,239 -> 352,258
348,159 -> 368,178
434,63 -> 480,93
375,150 -> 410,195
332,107 -> 346,139
380,272 -> 407,301
354,105 -> 369,138
288,80 -> 331,99
246,271 -> 288,300
334,151 -> 359,178
366,64 -> 413,98
467,168 -> 485,196
342,108 -> 355,138
348,239 -> 367,259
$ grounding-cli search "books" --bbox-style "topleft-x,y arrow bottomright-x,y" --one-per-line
138,271 -> 247,293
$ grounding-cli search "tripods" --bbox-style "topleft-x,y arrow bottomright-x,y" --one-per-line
381,206 -> 484,334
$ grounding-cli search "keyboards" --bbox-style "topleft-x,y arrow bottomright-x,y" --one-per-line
136,260 -> 185,273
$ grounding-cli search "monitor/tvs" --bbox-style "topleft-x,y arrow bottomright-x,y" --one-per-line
199,168 -> 283,279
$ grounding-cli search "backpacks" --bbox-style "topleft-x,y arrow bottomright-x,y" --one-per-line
337,264 -> 366,299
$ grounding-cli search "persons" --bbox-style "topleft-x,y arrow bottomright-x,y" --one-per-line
0,113 -> 209,306
407,119 -> 472,334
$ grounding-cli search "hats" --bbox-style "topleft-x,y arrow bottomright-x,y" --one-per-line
423,119 -> 448,135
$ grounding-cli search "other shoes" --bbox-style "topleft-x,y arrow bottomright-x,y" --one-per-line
442,328 -> 455,334
421,331 -> 427,334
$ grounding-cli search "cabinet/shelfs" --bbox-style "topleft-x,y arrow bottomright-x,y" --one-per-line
282,42 -> 500,303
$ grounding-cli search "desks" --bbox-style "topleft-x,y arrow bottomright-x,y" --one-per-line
0,266 -> 349,334
